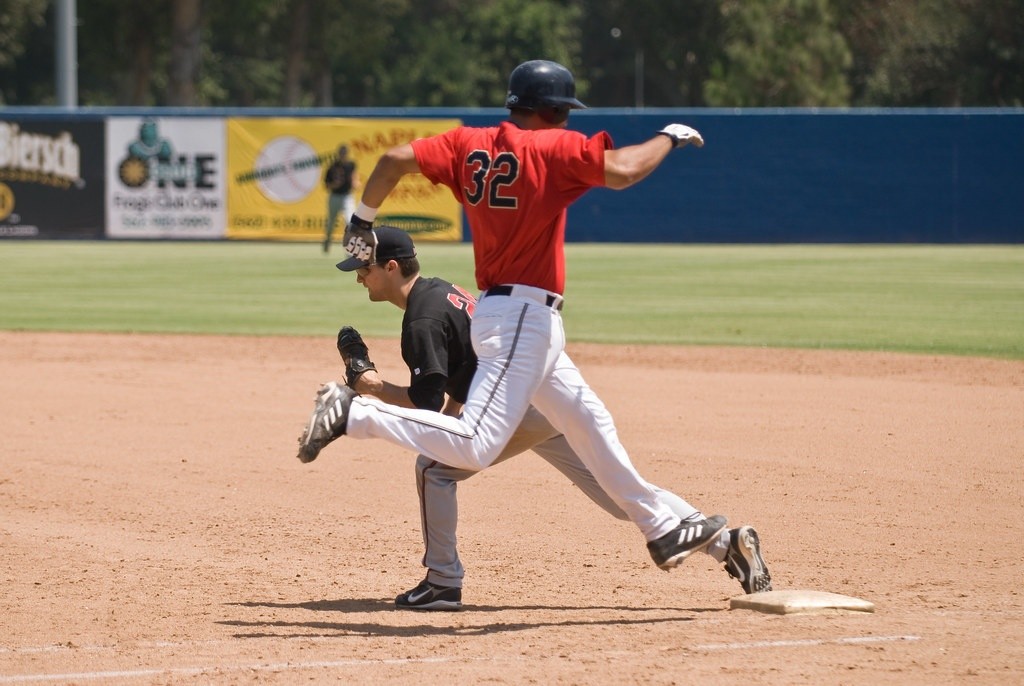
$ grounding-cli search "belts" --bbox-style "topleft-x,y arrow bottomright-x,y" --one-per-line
485,285 -> 564,310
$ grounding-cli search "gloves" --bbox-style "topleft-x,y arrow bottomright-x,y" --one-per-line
656,123 -> 705,148
343,213 -> 378,267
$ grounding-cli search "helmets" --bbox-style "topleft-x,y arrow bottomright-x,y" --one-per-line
505,59 -> 588,111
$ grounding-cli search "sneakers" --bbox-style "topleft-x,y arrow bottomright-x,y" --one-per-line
722,525 -> 773,594
394,579 -> 463,610
296,380 -> 359,463
645,511 -> 729,568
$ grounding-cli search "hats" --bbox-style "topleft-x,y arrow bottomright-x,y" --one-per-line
336,225 -> 418,271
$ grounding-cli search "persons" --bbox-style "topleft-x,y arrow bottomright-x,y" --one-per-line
334,225 -> 773,609
297,60 -> 728,571
323,143 -> 357,256
